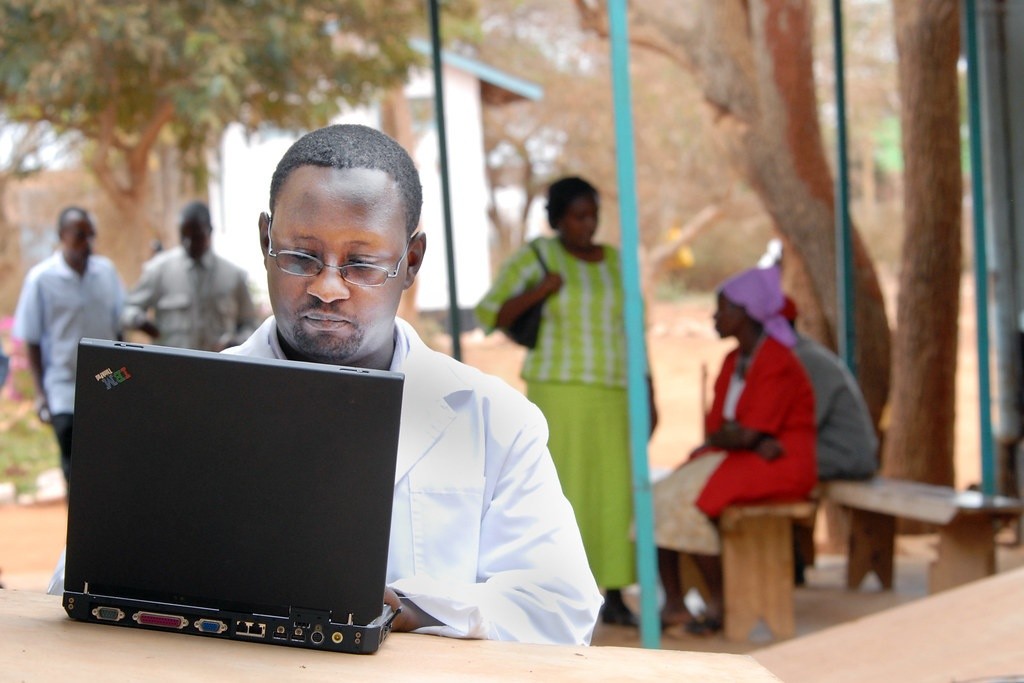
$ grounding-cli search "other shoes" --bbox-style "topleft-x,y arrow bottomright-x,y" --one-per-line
686,611 -> 726,639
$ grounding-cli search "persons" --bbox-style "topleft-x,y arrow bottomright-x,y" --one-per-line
126,200 -> 259,354
15,205 -> 126,483
652,266 -> 881,638
474,174 -> 658,629
219,124 -> 603,648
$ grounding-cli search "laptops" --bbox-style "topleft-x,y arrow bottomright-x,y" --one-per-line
62,336 -> 401,655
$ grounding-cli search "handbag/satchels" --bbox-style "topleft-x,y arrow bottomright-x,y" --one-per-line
500,242 -> 551,349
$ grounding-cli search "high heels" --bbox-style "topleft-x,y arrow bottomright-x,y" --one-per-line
602,605 -> 643,627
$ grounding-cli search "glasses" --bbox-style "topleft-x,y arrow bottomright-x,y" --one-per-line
268,216 -> 412,288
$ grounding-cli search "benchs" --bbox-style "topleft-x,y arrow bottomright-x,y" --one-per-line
677,504 -> 815,647
820,477 -> 1024,587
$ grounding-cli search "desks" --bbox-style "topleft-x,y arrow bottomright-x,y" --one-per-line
0,586 -> 781,683
